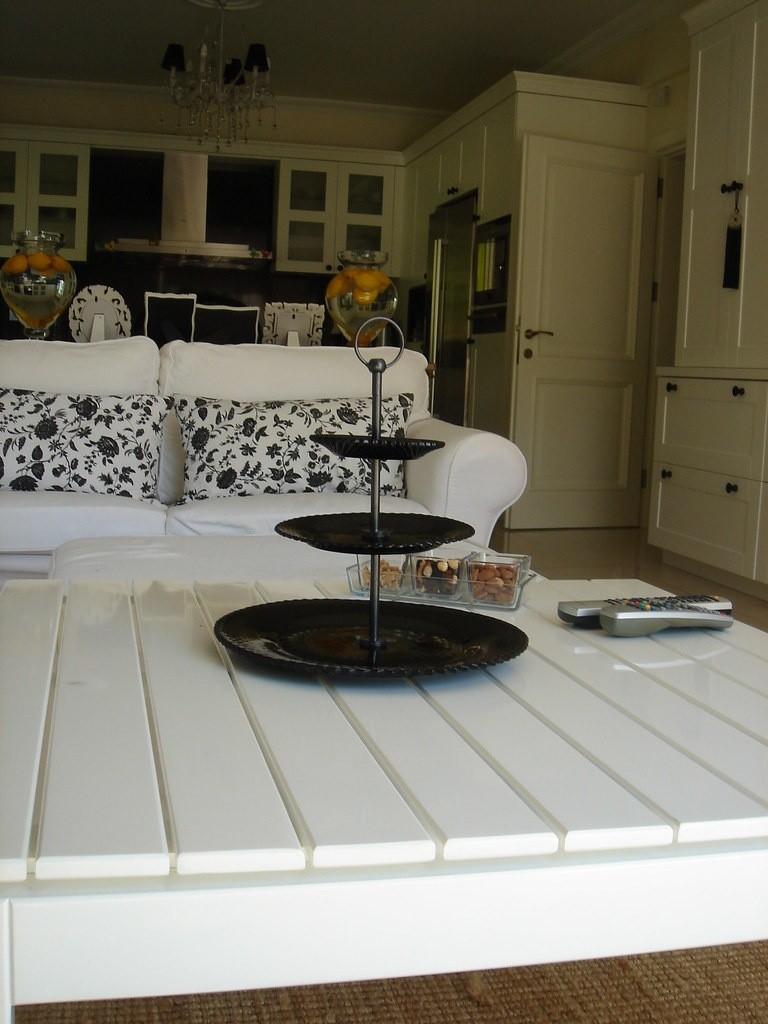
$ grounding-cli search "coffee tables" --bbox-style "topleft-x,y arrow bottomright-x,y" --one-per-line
0,576 -> 768,1024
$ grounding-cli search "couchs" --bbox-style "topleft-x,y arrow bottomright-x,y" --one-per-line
0,336 -> 529,584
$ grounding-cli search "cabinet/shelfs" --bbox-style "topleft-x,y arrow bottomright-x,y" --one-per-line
0,138 -> 90,262
406,92 -> 649,440
647,367 -> 768,581
275,158 -> 395,277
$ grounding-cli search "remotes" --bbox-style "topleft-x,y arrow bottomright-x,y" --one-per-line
558,595 -> 734,637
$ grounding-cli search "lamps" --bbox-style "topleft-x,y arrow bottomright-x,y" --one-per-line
159,0 -> 278,151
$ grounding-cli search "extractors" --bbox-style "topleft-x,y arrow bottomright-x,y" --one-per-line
105,238 -> 273,270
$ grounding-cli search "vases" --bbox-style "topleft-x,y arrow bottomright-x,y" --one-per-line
195,304 -> 261,345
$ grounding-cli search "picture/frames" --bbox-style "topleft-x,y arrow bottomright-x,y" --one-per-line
262,302 -> 325,347
144,291 -> 197,349
68,284 -> 132,343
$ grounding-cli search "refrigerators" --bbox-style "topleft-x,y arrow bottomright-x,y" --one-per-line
424,197 -> 476,426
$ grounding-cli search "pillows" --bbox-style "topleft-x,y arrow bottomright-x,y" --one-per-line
172,392 -> 415,506
0,387 -> 176,505
